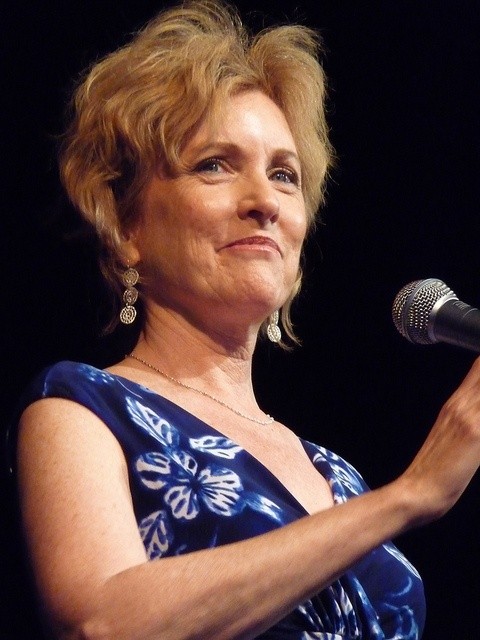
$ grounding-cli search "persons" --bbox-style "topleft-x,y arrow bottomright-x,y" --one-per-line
17,0 -> 480,640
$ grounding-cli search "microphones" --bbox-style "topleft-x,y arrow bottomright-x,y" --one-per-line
392,278 -> 480,353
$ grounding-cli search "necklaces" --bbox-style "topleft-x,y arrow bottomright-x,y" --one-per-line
124,351 -> 275,426
